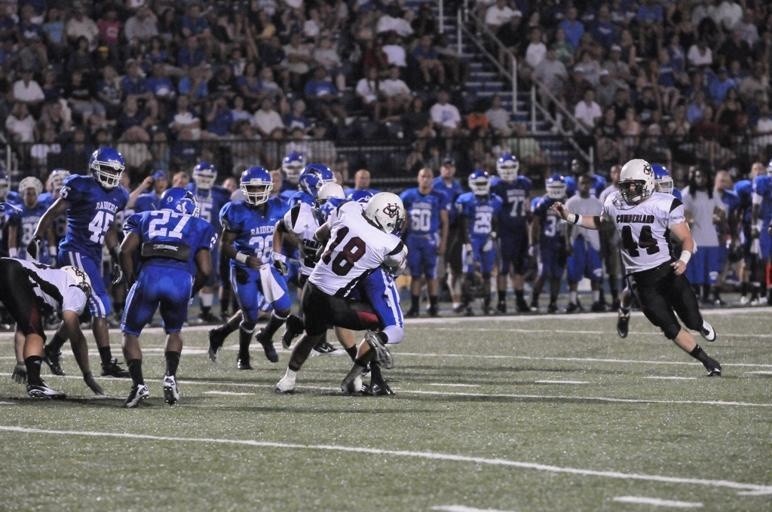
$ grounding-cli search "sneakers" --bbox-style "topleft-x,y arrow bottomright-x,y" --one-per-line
341,329 -> 396,397
699,320 -> 717,343
276,377 -> 297,394
616,307 -> 630,339
403,298 -> 620,318
14,348 -> 179,406
198,308 -> 223,325
209,329 -> 335,370
706,365 -> 722,376
697,295 -> 769,308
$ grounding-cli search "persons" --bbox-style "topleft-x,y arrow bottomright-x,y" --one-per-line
0,0 -> 770,319
615,162 -> 698,338
0,145 -> 409,408
553,158 -> 723,376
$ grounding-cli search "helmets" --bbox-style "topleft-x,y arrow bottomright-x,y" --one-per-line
468,170 -> 491,197
496,154 -> 519,182
545,174 -> 567,200
88,146 -> 127,189
239,152 -> 407,236
192,160 -> 218,189
18,177 -> 44,210
617,159 -> 674,207
158,186 -> 201,220
45,167 -> 71,200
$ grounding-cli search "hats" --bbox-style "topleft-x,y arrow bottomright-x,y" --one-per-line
441,157 -> 454,167
